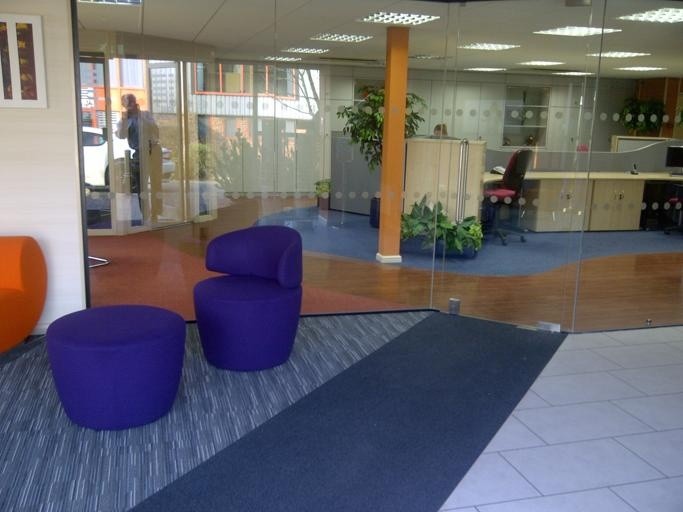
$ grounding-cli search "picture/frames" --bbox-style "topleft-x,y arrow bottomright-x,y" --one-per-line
0,12 -> 49,109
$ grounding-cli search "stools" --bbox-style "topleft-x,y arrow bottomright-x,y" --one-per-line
43,302 -> 189,434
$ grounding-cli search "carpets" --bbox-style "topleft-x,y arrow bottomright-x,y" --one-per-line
122,311 -> 572,511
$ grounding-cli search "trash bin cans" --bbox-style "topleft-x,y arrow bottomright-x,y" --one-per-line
370,197 -> 380,228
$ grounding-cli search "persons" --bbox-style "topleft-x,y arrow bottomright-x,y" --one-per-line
115,94 -> 163,224
434,123 -> 447,136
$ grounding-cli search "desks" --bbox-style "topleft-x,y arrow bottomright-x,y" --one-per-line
588,171 -> 682,233
482,170 -> 592,235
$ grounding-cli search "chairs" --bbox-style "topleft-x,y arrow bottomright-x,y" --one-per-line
478,146 -> 534,247
192,223 -> 304,374
0,233 -> 48,356
661,182 -> 682,237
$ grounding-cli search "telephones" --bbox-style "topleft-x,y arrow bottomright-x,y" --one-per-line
631,163 -> 638,175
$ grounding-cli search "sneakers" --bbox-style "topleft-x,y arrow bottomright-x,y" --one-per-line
142,213 -> 157,224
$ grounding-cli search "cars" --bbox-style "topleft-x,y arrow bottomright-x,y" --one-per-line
82,124 -> 176,194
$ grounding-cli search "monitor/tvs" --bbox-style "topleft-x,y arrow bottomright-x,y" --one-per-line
666,146 -> 683,177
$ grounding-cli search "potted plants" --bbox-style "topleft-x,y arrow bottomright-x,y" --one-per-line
396,193 -> 483,259
314,177 -> 331,210
